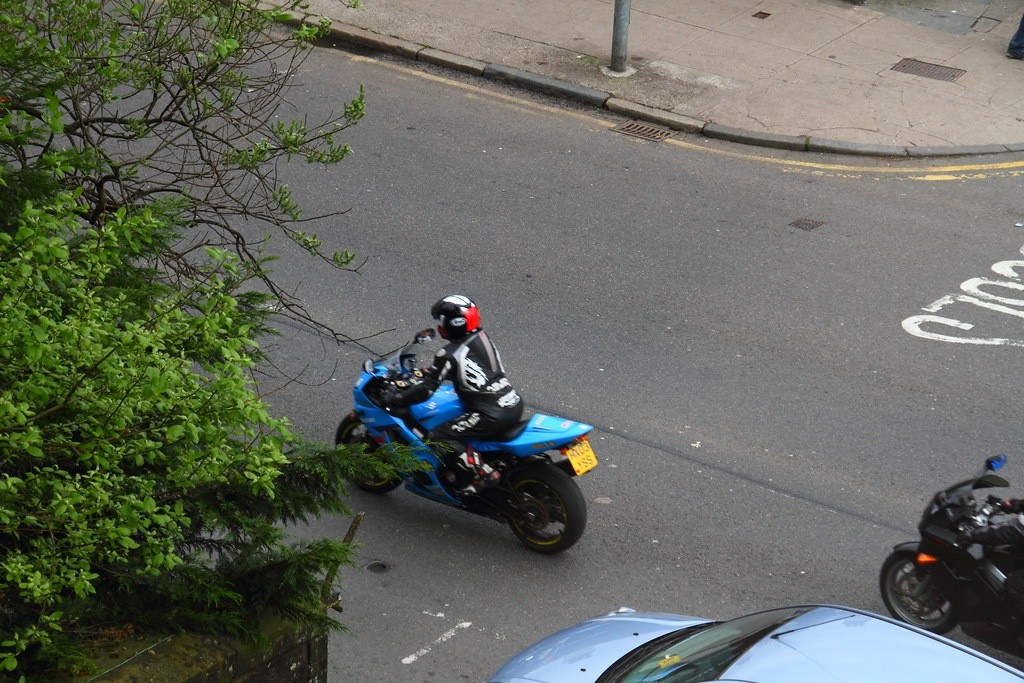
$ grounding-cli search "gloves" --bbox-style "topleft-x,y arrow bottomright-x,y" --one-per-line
376,387 -> 394,404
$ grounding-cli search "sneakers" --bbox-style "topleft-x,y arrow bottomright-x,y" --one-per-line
453,445 -> 501,497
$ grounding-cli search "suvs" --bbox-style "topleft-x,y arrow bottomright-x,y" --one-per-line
487,606 -> 1023,683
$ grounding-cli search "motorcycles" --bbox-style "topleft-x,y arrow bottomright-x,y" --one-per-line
334,328 -> 597,557
879,452 -> 1023,661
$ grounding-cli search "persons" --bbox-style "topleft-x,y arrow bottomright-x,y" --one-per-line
379,295 -> 523,493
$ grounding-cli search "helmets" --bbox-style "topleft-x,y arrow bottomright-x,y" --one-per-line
431,294 -> 481,341
